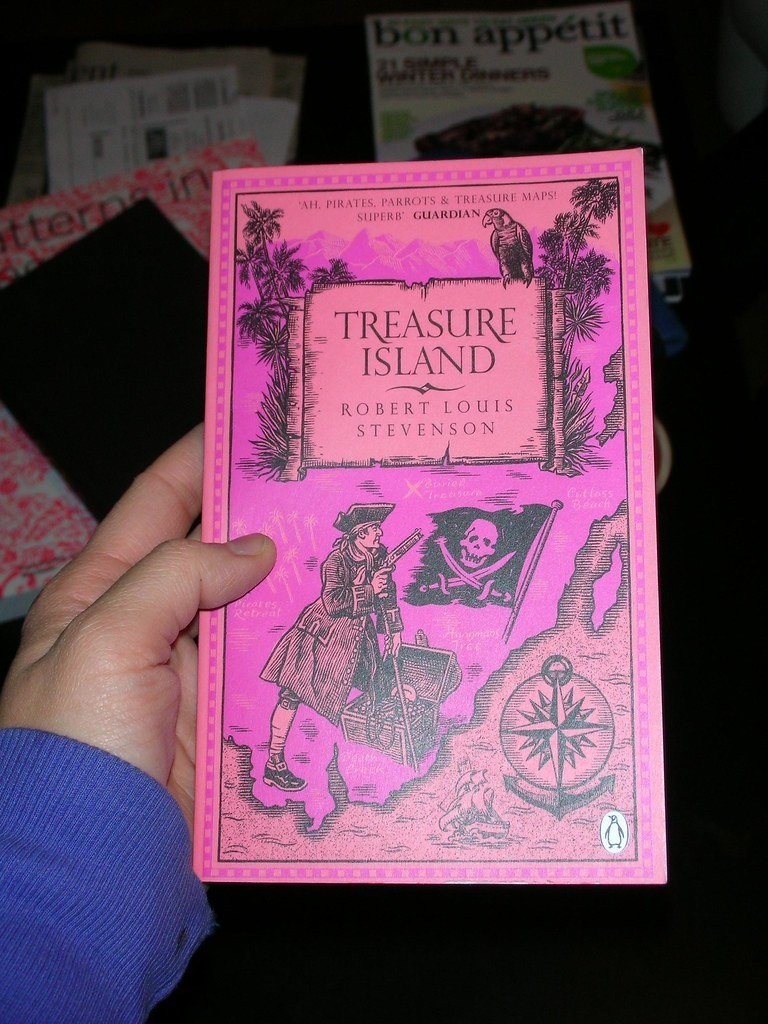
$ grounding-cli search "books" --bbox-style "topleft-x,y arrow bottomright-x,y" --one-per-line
0,137 -> 270,625
192,147 -> 667,888
366,5 -> 691,292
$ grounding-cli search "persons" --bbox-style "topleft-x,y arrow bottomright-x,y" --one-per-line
0,423 -> 276,1024
